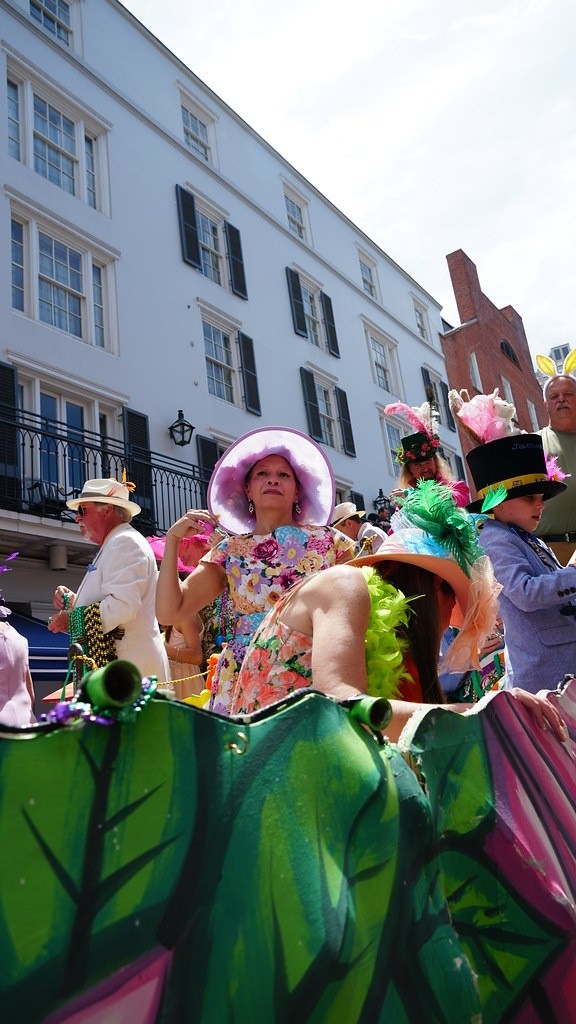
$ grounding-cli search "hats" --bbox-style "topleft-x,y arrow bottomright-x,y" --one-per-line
146,521 -> 213,574
207,426 -> 338,533
398,430 -> 438,463
341,527 -> 474,630
464,435 -> 566,514
67,479 -> 142,517
328,502 -> 366,527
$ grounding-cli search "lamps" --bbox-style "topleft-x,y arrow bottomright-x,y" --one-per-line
48,545 -> 68,570
373,488 -> 391,510
167,409 -> 196,447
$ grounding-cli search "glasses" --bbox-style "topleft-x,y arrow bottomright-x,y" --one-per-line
73,505 -> 91,515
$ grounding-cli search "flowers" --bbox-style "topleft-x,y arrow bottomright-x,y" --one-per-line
205,522 -> 357,715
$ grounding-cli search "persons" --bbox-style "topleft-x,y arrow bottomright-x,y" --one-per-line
388,429 -> 470,512
151,428 -> 359,719
365,505 -> 391,534
462,429 -> 576,695
47,477 -> 175,696
326,501 -> 390,559
176,508 -> 240,690
228,522 -> 570,744
151,540 -> 207,702
0,585 -> 39,734
516,372 -> 576,543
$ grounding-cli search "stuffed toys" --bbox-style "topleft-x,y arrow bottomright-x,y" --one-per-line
456,388 -> 573,481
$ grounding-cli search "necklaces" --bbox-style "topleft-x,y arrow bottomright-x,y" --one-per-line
205,584 -> 236,653
47,590 -> 118,698
